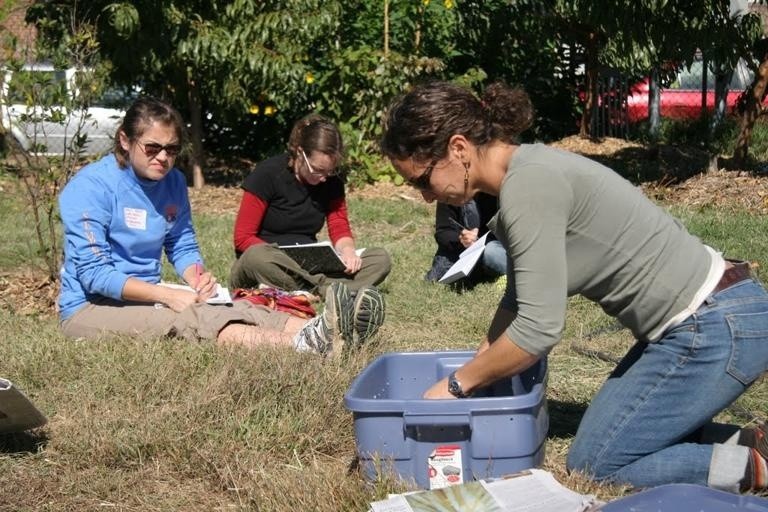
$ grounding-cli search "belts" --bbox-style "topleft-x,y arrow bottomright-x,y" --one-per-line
709,258 -> 753,297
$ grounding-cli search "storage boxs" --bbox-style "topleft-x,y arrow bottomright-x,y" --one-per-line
344,350 -> 550,493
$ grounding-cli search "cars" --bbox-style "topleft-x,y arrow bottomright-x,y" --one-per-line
576,52 -> 768,144
0,63 -> 227,156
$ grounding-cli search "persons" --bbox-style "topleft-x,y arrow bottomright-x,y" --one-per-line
56,97 -> 388,358
227,114 -> 393,304
380,83 -> 767,495
423,189 -> 507,289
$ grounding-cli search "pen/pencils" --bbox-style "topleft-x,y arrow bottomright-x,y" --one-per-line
448,218 -> 479,240
296,242 -> 299,246
195,260 -> 203,289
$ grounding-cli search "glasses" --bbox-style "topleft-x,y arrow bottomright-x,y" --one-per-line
302,150 -> 337,177
405,160 -> 437,193
131,136 -> 182,155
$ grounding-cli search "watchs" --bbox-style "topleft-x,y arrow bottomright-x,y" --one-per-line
448,371 -> 469,398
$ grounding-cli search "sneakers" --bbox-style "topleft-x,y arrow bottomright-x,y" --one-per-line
352,286 -> 386,352
299,280 -> 356,366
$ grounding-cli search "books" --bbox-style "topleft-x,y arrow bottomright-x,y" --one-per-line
438,228 -> 490,286
277,241 -> 366,276
152,281 -> 233,309
